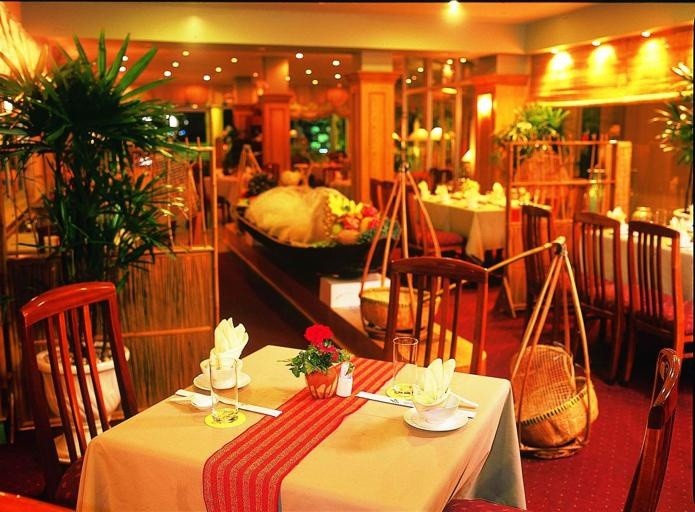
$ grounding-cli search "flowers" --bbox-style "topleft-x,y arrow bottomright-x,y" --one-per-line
276,323 -> 354,379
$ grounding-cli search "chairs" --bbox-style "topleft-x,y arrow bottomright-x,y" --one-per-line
15,280 -> 139,511
381,255 -> 491,376
438,346 -> 682,512
519,203 -> 583,369
617,219 -> 695,402
365,176 -> 465,295
567,208 -> 623,386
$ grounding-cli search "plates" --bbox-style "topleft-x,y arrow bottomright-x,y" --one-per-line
193,372 -> 250,390
404,407 -> 468,432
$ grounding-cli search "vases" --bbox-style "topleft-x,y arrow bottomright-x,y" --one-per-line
304,363 -> 340,400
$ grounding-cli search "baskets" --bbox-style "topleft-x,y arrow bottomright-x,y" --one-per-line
509,344 -> 599,448
359,287 -> 441,330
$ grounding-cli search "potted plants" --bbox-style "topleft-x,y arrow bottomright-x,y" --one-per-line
1,27 -> 203,466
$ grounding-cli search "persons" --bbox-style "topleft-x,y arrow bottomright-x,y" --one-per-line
239,125 -> 263,167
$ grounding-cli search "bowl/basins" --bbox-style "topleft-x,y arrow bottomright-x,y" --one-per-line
199,358 -> 244,386
413,398 -> 460,424
192,396 -> 218,410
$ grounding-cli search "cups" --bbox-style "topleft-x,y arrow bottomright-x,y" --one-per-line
210,359 -> 238,424
336,378 -> 354,397
392,337 -> 419,395
655,209 -> 666,227
